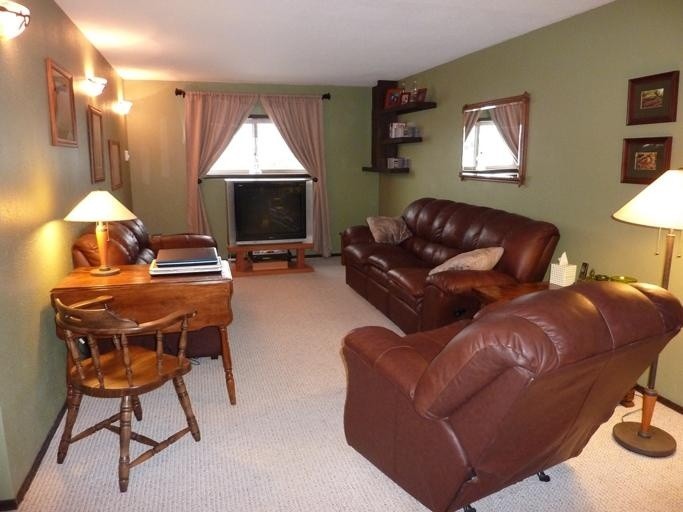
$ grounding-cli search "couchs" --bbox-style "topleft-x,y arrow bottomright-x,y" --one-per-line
69,210 -> 225,365
337,194 -> 562,336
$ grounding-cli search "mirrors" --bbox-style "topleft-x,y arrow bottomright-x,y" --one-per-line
458,91 -> 530,188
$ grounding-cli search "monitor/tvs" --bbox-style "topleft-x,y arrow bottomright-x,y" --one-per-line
225,178 -> 314,245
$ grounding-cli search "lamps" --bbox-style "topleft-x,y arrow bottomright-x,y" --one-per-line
61,192 -> 143,278
79,73 -> 107,99
111,99 -> 133,118
608,164 -> 683,462
0,0 -> 34,44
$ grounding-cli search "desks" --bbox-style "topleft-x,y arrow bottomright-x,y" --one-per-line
49,258 -> 239,414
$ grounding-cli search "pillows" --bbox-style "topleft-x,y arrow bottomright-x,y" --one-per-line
426,246 -> 505,281
365,209 -> 413,245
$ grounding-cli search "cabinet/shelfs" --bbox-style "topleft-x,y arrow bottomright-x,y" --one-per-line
360,78 -> 438,175
228,241 -> 316,278
469,281 -> 563,310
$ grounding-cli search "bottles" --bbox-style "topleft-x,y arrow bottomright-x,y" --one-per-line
399,79 -> 418,105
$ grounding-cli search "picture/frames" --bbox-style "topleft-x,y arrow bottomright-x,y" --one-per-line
616,134 -> 675,188
622,70 -> 681,128
45,52 -> 81,151
108,136 -> 124,192
84,105 -> 107,185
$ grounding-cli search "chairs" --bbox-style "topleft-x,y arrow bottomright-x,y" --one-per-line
52,296 -> 205,493
337,268 -> 683,511
